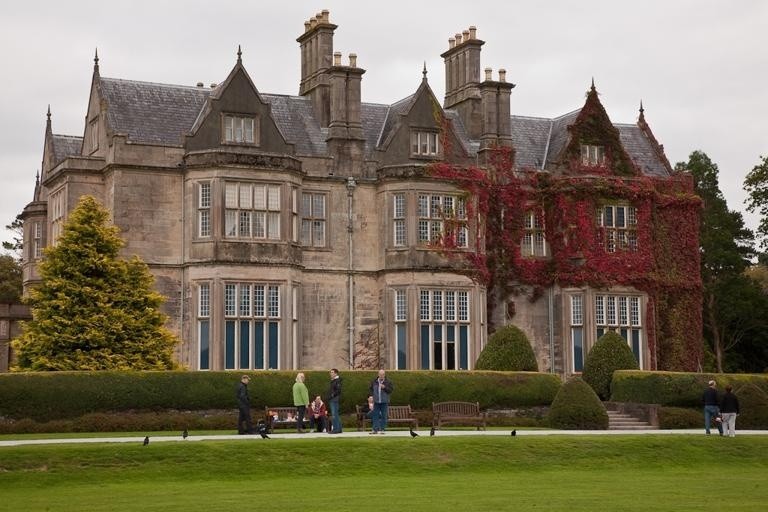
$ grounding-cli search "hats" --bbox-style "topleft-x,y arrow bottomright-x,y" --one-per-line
242,376 -> 250,380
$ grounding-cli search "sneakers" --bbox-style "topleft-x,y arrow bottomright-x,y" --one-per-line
368,430 -> 376,434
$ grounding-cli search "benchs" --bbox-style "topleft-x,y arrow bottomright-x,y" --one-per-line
264,406 -> 331,434
356,401 -> 486,431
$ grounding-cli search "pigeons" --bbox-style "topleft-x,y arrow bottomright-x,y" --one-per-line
409,427 -> 420,437
510,428 -> 516,437
430,426 -> 435,437
260,430 -> 271,440
182,428 -> 189,441
143,435 -> 149,446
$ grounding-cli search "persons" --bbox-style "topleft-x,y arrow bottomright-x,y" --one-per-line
717,383 -> 741,438
292,372 -> 310,433
236,374 -> 257,434
361,393 -> 382,419
306,393 -> 329,432
367,369 -> 394,435
324,368 -> 343,433
700,380 -> 725,436
